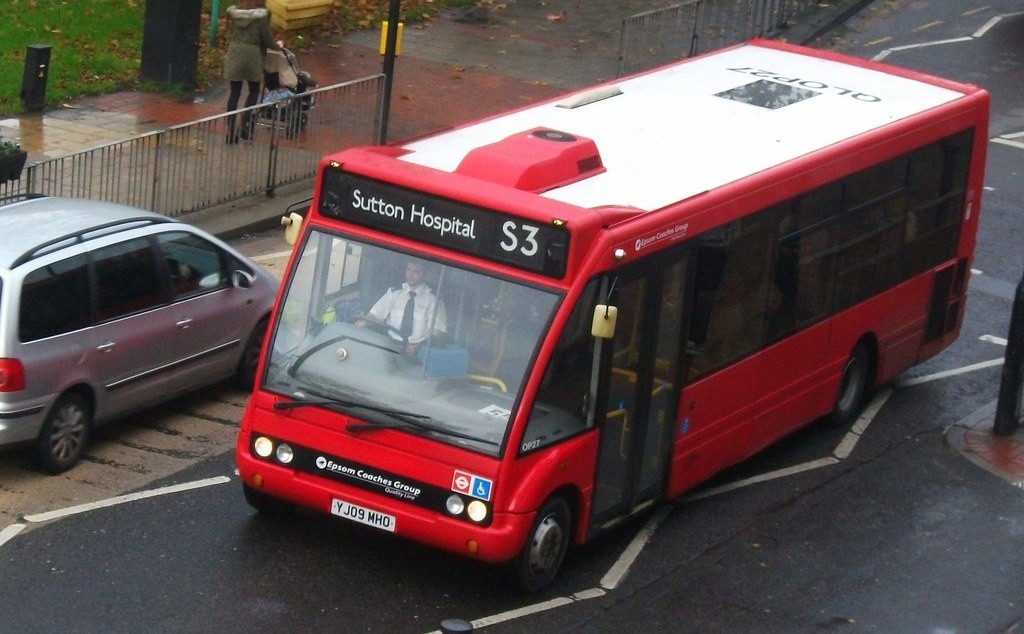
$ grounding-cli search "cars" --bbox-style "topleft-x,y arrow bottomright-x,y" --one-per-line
0,191 -> 284,471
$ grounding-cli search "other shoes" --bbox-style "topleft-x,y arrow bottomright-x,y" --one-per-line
241,127 -> 253,140
226,132 -> 239,144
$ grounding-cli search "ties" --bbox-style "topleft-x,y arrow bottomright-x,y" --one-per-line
400,291 -> 416,338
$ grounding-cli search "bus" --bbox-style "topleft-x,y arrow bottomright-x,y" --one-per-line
232,38 -> 996,596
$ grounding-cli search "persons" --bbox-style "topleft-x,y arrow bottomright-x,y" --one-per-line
136,244 -> 191,294
298,74 -> 319,87
354,261 -> 447,357
223,0 -> 283,144
708,189 -> 919,367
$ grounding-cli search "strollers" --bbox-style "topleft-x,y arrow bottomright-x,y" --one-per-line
243,45 -> 316,132
